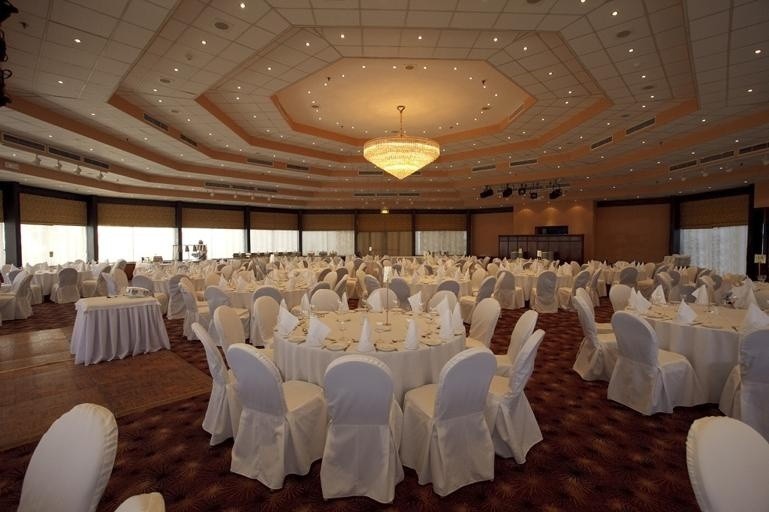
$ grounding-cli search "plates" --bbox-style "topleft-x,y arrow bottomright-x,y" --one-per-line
326,343 -> 348,351
292,300 -> 466,329
626,297 -> 751,332
422,339 -> 444,348
289,336 -> 305,343
375,343 -> 397,353
453,331 -> 465,336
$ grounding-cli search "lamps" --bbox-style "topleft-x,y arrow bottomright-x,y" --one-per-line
480,184 -> 562,200
364,107 -> 440,181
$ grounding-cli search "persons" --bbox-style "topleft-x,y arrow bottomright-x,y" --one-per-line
198,239 -> 207,261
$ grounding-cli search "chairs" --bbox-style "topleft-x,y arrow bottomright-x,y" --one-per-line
570,296 -> 618,378
18,403 -> 118,512
225,343 -> 326,490
485,328 -> 545,463
493,309 -> 539,374
117,491 -> 169,511
719,328 -> 768,435
604,310 -> 709,416
686,415 -> 768,511
1,250 -> 767,365
397,347 -> 497,494
319,354 -> 404,503
189,322 -> 243,446
